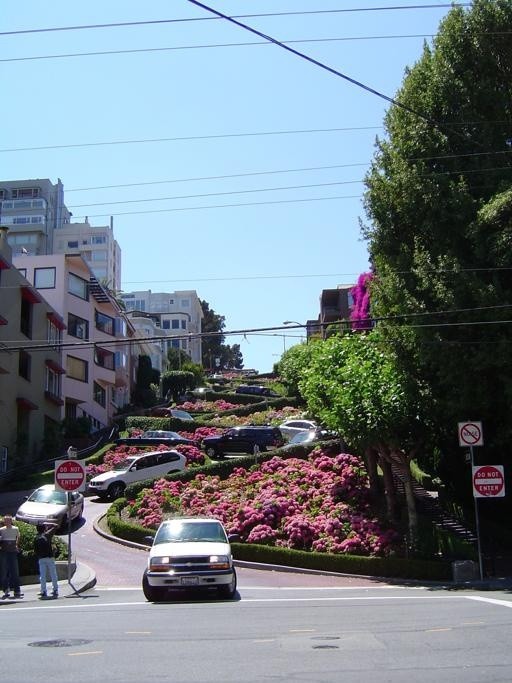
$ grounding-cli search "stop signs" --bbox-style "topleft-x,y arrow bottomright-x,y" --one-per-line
472,464 -> 506,497
53,458 -> 86,493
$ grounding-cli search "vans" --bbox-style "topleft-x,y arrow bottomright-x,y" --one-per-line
237,385 -> 283,398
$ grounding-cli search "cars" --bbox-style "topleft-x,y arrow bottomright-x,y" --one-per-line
209,374 -> 223,379
192,387 -> 214,392
142,517 -> 237,601
144,430 -> 190,440
287,431 -> 329,447
14,482 -> 85,535
279,420 -> 340,435
150,409 -> 194,420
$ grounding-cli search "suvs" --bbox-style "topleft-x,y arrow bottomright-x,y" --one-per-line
87,447 -> 188,502
202,425 -> 284,457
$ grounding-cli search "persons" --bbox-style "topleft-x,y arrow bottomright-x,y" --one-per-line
34,522 -> 61,596
0,515 -> 24,599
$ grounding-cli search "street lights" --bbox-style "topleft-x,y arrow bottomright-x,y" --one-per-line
284,320 -> 309,345
274,334 -> 286,353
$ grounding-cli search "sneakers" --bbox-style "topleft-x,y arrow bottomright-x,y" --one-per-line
37,590 -> 59,596
2,592 -> 24,597
